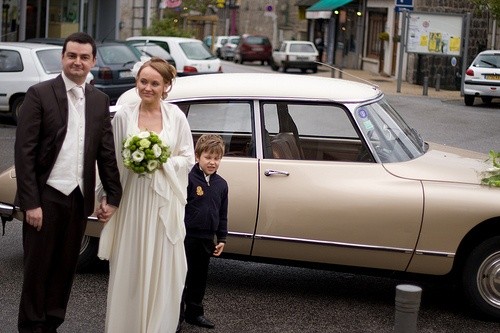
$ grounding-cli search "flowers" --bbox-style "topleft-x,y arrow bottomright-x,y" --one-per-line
129,56 -> 151,78
120,128 -> 172,179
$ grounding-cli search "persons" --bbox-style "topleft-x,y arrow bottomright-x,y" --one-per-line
97,57 -> 195,333
176,134 -> 228,333
315,31 -> 324,62
14,33 -> 123,333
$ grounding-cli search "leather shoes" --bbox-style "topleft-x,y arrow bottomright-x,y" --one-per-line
184,314 -> 215,329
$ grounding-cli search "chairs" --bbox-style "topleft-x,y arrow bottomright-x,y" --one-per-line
275,133 -> 302,160
270,138 -> 292,160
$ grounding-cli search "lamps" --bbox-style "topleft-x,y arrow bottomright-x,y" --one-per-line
120,18 -> 125,30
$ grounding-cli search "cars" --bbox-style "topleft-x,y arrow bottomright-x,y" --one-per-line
271,39 -> 320,73
18,37 -> 177,106
0,40 -> 94,125
234,33 -> 273,66
463,50 -> 500,106
204,35 -> 240,60
0,70 -> 500,323
124,35 -> 224,76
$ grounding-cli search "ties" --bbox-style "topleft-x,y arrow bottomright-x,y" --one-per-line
71,85 -> 84,115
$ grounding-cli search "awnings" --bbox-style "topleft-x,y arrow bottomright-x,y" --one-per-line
305,0 -> 353,19
294,0 -> 320,6
